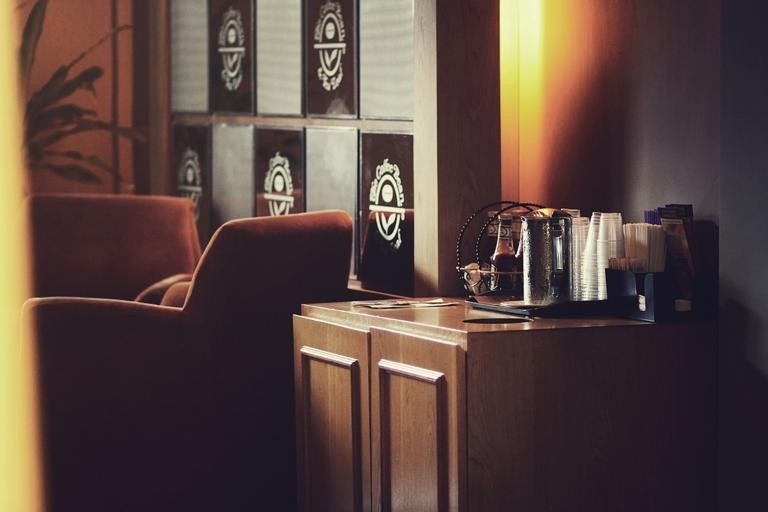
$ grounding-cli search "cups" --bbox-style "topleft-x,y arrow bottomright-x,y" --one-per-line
564,210 -> 625,302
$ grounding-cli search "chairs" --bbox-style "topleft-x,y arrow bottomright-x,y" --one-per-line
26,192 -> 200,302
22,211 -> 353,512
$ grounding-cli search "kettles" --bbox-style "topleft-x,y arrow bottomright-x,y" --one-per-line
516,207 -> 571,305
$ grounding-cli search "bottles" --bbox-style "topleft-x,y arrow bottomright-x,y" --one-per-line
491,213 -> 516,291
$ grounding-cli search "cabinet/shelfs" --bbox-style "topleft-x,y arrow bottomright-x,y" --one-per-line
292,287 -> 716,512
